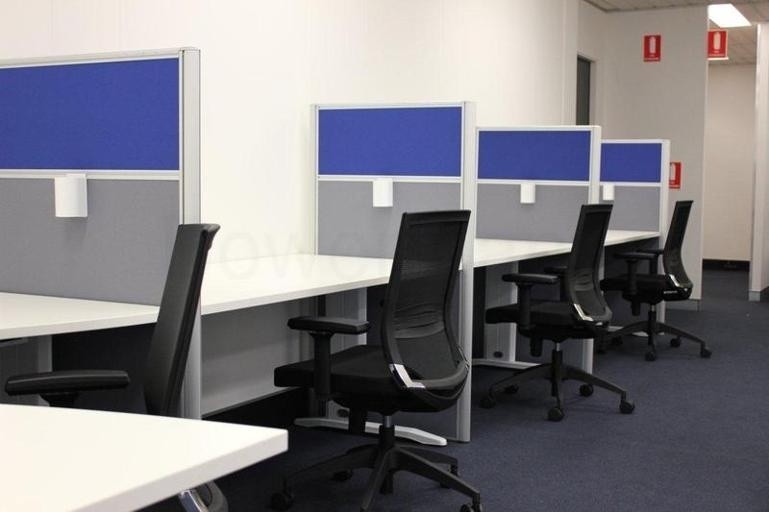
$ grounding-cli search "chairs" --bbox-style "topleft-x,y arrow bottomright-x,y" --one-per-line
480,200 -> 635,423
268,209 -> 477,512
600,201 -> 714,361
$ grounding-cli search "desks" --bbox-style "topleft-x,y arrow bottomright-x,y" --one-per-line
467,126 -> 600,378
599,139 -> 671,336
1,48 -> 204,512
2,402 -> 288,512
200,98 -> 472,445
